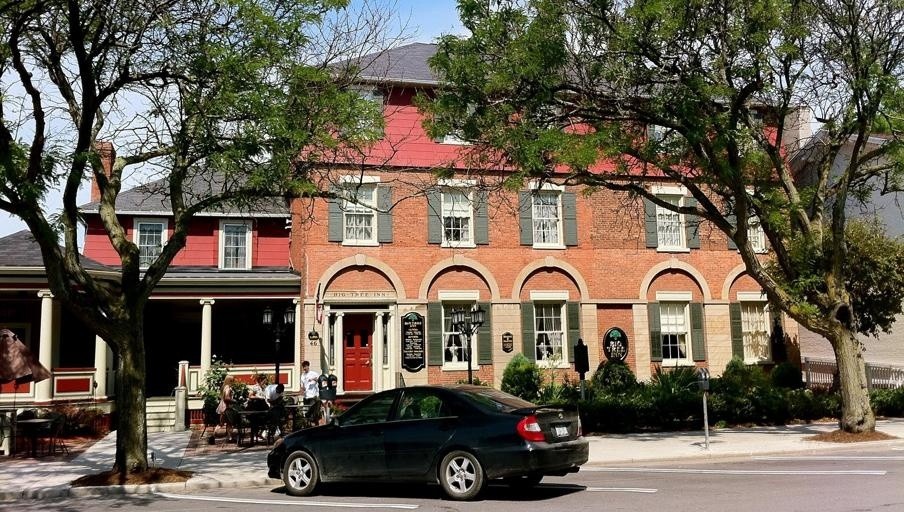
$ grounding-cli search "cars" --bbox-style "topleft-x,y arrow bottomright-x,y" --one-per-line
266,382 -> 590,501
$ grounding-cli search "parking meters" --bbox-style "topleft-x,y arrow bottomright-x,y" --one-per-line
697,366 -> 713,451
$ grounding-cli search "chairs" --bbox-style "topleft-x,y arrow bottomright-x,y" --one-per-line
201,397 -> 323,448
0,409 -> 70,456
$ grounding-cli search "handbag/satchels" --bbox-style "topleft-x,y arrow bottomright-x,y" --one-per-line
216,399 -> 227,414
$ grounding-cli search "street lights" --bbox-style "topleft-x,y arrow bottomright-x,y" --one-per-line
450,304 -> 487,384
261,305 -> 296,388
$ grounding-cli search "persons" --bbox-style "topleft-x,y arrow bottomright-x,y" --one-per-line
289,360 -> 321,426
205,374 -> 238,444
262,381 -> 287,441
246,373 -> 268,443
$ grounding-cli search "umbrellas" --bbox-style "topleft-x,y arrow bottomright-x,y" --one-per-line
0,326 -> 51,383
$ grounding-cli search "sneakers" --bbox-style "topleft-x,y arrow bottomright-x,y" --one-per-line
208,436 -> 215,445
